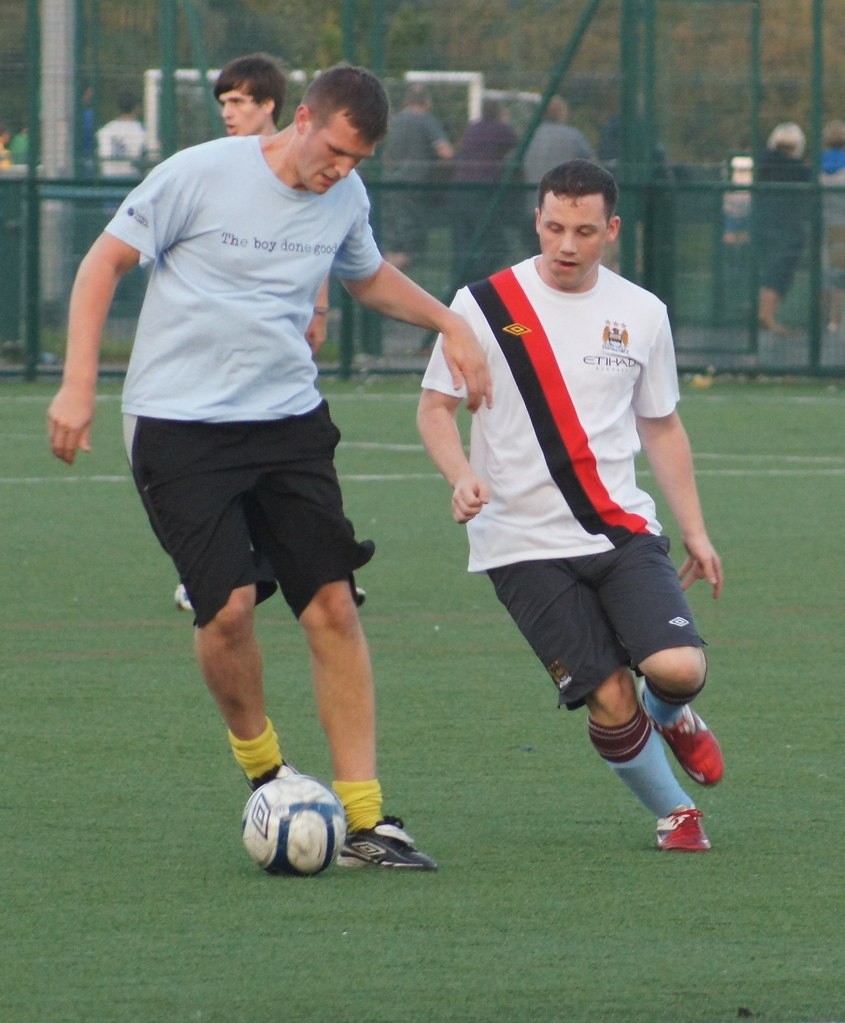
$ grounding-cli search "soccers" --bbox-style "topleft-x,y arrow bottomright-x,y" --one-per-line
240,773 -> 349,877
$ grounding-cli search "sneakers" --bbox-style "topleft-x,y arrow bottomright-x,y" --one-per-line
336,815 -> 436,870
639,680 -> 723,788
242,755 -> 301,795
655,804 -> 714,851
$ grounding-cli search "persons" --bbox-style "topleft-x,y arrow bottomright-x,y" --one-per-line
520,93 -> 597,255
9,125 -> 37,164
382,83 -> 457,269
449,97 -> 522,267
0,124 -> 12,171
81,73 -> 97,157
816,116 -> 845,329
94,87 -> 161,222
748,123 -> 820,334
599,91 -> 691,277
46,64 -> 496,874
172,52 -> 367,610
415,160 -> 728,851
